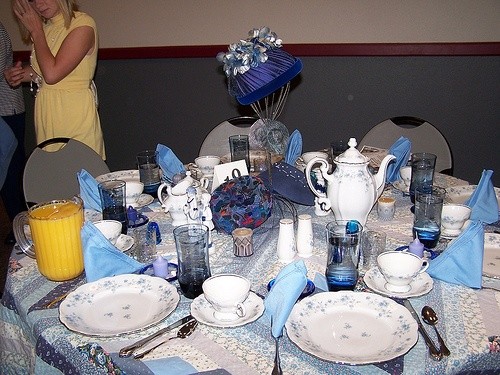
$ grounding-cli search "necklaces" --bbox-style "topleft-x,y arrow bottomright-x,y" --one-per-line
29,26 -> 62,101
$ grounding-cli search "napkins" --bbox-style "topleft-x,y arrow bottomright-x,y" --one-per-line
465,169 -> 500,225
284,129 -> 302,167
386,137 -> 411,184
263,260 -> 308,338
155,144 -> 186,183
76,169 -> 102,213
426,217 -> 483,291
79,220 -> 146,283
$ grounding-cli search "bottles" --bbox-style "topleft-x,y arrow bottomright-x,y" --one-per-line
275,214 -> 313,264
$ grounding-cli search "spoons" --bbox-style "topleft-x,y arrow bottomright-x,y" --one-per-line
134,320 -> 198,359
421,306 -> 450,356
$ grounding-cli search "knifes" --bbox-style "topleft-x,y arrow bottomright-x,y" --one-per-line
403,300 -> 442,361
119,314 -> 193,358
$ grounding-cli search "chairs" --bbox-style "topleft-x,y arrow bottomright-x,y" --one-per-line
22,138 -> 110,210
359,116 -> 454,177
198,116 -> 260,163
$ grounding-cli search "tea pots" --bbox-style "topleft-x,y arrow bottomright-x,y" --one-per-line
306,138 -> 396,227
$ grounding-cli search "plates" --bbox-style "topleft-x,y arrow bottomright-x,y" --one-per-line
363,265 -> 434,299
115,233 -> 135,252
444,184 -> 500,209
136,194 -> 154,208
58,273 -> 180,338
95,169 -> 144,195
190,292 -> 265,328
284,289 -> 419,366
448,232 -> 500,291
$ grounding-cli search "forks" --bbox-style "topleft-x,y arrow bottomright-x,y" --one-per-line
270,315 -> 284,375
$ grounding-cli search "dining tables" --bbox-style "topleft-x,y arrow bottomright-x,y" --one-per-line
4,164 -> 500,375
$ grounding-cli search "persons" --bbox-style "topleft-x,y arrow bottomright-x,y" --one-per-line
4,0 -> 106,161
0,23 -> 27,245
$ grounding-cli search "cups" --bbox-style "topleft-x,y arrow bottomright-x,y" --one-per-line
330,141 -> 350,174
324,219 -> 363,292
414,185 -> 446,250
409,153 -> 438,214
377,197 -> 396,219
440,203 -> 472,237
376,251 -> 430,293
400,166 -> 412,192
201,273 -> 251,317
16,134 -> 252,299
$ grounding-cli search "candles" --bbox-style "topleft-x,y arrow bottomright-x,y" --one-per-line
127,205 -> 137,222
409,232 -> 424,259
152,253 -> 169,278
